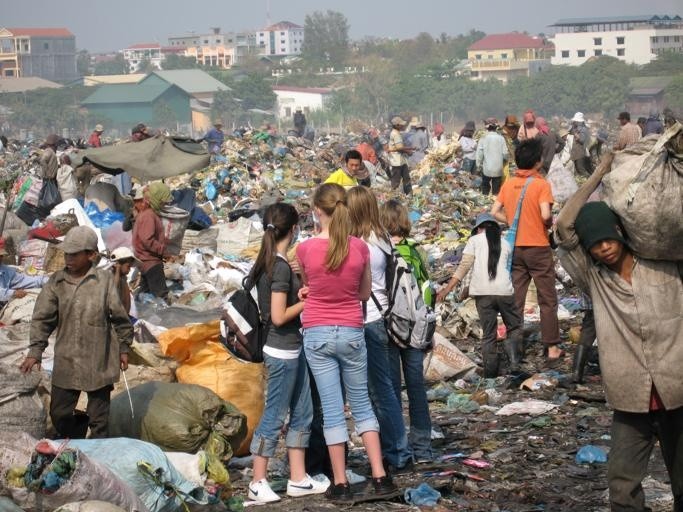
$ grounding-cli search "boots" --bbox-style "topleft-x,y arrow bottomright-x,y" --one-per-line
503,338 -> 521,375
483,354 -> 500,378
573,344 -> 589,382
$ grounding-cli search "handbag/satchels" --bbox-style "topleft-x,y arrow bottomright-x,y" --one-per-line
503,230 -> 516,272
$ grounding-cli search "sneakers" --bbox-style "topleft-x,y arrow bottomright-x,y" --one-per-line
248,481 -> 281,503
373,471 -> 397,495
287,473 -> 331,497
325,481 -> 352,501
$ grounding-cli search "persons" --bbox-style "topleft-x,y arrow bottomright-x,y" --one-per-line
133,182 -> 173,300
357,113 -> 428,194
40,123 -> 224,199
295,109 -> 314,142
433,107 -> 682,511
18,225 -> 135,437
108,245 -> 144,316
239,147 -> 433,504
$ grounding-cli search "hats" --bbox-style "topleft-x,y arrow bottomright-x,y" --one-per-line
110,247 -> 143,263
56,226 -> 98,254
616,112 -> 630,120
96,124 -> 104,132
409,117 -> 421,126
471,214 -> 501,235
559,129 -> 570,137
391,117 -> 407,125
571,112 -> 585,122
47,134 -> 60,144
214,119 -> 223,125
574,201 -> 626,251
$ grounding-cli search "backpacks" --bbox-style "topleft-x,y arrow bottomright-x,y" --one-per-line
218,257 -> 293,362
370,238 -> 436,350
